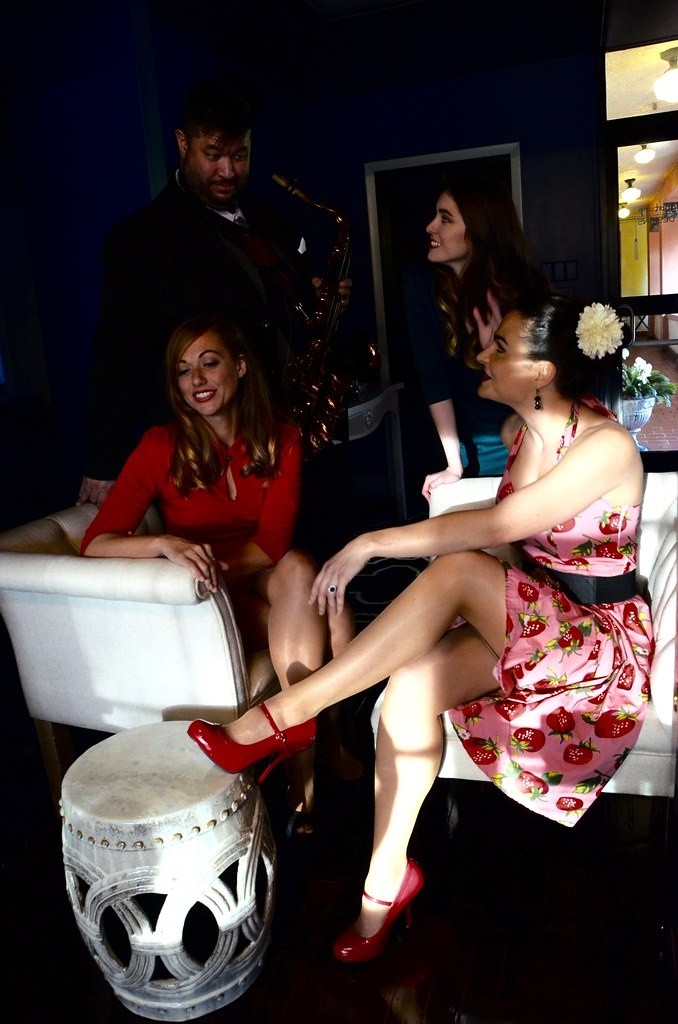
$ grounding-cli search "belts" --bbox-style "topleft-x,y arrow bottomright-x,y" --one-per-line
546,569 -> 638,606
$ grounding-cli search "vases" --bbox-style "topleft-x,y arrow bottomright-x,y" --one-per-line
614,395 -> 657,452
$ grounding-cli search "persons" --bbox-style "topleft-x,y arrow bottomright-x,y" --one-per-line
106,88 -> 352,443
414,173 -> 569,500
81,313 -> 365,846
184,291 -> 654,962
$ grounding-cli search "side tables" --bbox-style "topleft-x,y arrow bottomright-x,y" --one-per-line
57,716 -> 283,1024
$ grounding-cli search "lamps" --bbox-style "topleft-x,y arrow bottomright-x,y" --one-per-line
634,143 -> 656,164
617,202 -> 630,219
620,177 -> 641,204
652,47 -> 678,105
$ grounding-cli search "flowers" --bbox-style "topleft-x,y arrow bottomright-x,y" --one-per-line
618,356 -> 678,408
573,303 -> 626,360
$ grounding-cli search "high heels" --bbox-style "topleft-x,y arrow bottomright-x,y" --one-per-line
335,859 -> 424,961
188,702 -> 318,785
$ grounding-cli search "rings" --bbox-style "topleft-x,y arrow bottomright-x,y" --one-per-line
328,586 -> 336,595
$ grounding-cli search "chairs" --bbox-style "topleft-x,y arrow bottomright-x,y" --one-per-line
0,497 -> 281,804
367,470 -> 675,801
